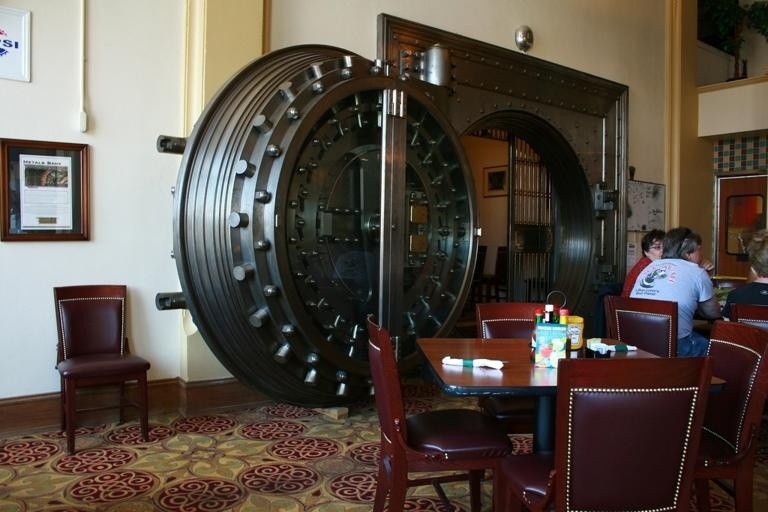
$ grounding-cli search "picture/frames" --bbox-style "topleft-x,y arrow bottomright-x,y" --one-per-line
0,138 -> 91,241
482,165 -> 508,197
0,3 -> 32,81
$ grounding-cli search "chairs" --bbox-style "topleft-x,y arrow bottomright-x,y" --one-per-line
477,247 -> 508,303
603,294 -> 678,359
476,303 -> 563,478
493,355 -> 716,512
693,319 -> 768,512
731,302 -> 768,328
367,313 -> 513,512
54,285 -> 151,455
462,245 -> 487,318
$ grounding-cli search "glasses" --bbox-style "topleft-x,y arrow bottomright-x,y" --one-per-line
648,245 -> 664,250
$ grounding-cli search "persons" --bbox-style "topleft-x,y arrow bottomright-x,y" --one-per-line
623,228 -> 664,297
722,229 -> 768,322
629,227 -> 722,357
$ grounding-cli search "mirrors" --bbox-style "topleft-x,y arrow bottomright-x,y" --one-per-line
724,194 -> 765,256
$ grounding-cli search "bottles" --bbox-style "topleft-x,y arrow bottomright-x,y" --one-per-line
560,309 -> 571,358
554,307 -> 560,324
568,316 -> 584,350
529,306 -> 544,362
543,304 -> 554,323
